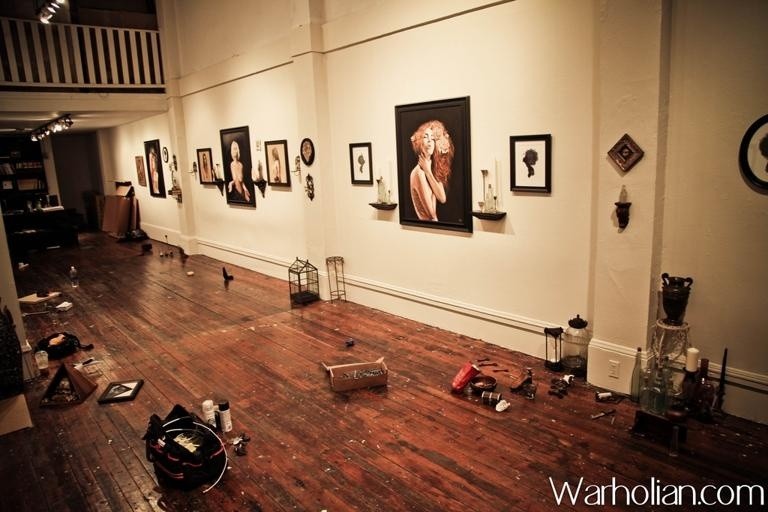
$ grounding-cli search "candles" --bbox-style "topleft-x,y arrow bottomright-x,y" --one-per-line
686,347 -> 700,373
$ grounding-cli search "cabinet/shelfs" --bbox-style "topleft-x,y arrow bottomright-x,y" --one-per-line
1,134 -> 48,209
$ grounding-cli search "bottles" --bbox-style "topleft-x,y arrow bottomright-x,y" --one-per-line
69,266 -> 78,288
562,314 -> 589,368
629,347 -> 644,404
201,400 -> 233,432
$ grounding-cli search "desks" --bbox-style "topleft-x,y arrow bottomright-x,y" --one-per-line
5,208 -> 78,259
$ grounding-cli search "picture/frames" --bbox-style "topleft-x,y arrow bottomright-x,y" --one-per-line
144,138 -> 171,200
394,95 -> 475,233
506,133 -> 553,195
195,125 -> 257,208
94,377 -> 144,404
347,142 -> 375,185
263,140 -> 296,188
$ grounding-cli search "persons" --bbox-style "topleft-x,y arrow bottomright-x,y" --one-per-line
149,144 -> 160,194
410,120 -> 455,221
228,140 -> 250,202
201,154 -> 211,181
271,148 -> 280,181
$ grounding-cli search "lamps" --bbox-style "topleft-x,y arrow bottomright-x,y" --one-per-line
29,112 -> 73,147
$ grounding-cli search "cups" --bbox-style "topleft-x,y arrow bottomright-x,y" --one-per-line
35,350 -> 50,379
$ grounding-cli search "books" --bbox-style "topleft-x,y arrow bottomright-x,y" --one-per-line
16,162 -> 42,169
3,179 -> 45,190
0,163 -> 16,175
